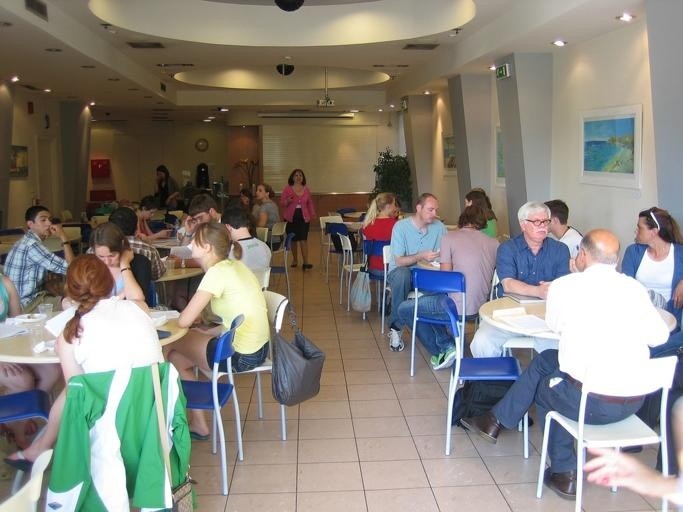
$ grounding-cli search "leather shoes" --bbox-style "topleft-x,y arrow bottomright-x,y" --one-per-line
459,413 -> 503,444
543,466 -> 578,499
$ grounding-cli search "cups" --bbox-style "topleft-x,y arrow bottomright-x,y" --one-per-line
166,259 -> 176,275
39,302 -> 55,316
29,327 -> 45,353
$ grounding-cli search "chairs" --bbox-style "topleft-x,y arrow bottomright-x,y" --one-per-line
325,222 -> 364,287
337,231 -> 367,311
271,221 -> 288,266
536,355 -> 679,512
410,267 -> 467,386
194,289 -> 289,441
255,226 -> 269,243
362,240 -> 390,320
319,216 -> 344,271
171,314 -> 244,495
381,245 -> 424,336
149,281 -> 156,309
0,388 -> 48,424
439,295 -> 530,459
271,232 -> 296,303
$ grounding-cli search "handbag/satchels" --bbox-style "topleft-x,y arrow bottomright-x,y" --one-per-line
349,259 -> 373,313
151,360 -> 200,512
452,381 -> 515,426
268,308 -> 326,407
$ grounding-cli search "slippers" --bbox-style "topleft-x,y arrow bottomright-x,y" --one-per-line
187,422 -> 212,441
4,451 -> 35,474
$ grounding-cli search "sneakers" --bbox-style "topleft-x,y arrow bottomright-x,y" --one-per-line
429,354 -> 437,366
432,345 -> 457,371
386,329 -> 406,353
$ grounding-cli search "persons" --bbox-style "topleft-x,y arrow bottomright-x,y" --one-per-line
55,223 -> 153,310
234,189 -> 258,221
461,230 -> 671,501
221,202 -> 272,291
362,192 -> 397,316
163,221 -> 269,441
397,205 -> 501,370
153,164 -> 180,207
544,200 -> 583,272
85,206 -> 167,313
166,190 -> 187,212
279,169 -> 316,272
254,183 -> 281,252
392,196 -> 400,221
387,192 -> 447,353
165,193 -> 221,327
469,201 -> 571,429
1,266 -> 62,450
133,195 -> 174,245
463,188 -> 498,239
583,396 -> 683,512
2,205 -> 74,314
620,346 -> 683,479
2,254 -> 167,474
621,207 -> 683,356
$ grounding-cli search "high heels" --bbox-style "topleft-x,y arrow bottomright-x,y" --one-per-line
290,259 -> 299,268
302,262 -> 313,270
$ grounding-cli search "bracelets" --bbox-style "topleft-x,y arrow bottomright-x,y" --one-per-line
121,267 -> 132,273
61,241 -> 70,247
184,232 -> 193,238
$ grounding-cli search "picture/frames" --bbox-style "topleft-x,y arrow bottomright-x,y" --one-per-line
491,121 -> 507,188
576,102 -> 641,189
440,131 -> 459,174
9,145 -> 30,179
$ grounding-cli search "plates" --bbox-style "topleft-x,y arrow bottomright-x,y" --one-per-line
14,313 -> 45,323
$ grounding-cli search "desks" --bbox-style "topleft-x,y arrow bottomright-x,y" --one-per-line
156,266 -> 203,306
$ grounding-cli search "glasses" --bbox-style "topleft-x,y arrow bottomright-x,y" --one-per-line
644,207 -> 661,230
190,215 -> 208,225
524,218 -> 554,227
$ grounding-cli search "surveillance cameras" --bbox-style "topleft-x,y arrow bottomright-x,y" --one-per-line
316,98 -> 326,107
325,99 -> 335,108
